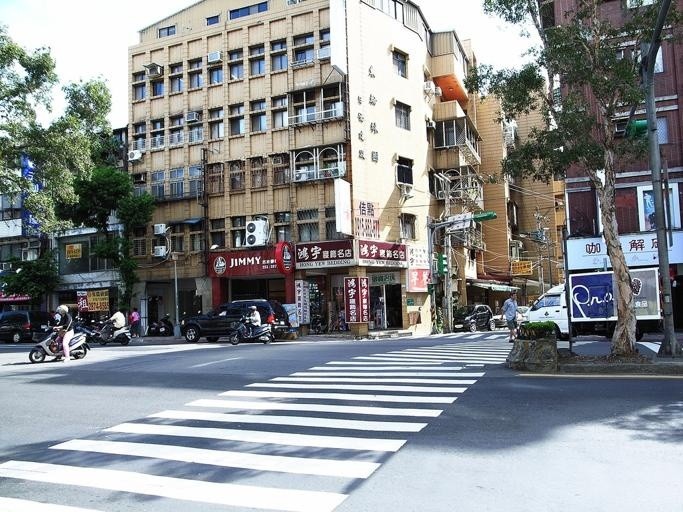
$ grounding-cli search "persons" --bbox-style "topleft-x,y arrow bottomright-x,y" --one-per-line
330,311 -> 346,333
129,307 -> 141,337
240,306 -> 262,338
50,305 -> 75,362
500,292 -> 524,343
104,308 -> 126,339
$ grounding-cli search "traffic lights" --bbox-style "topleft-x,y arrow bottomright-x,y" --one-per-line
437,252 -> 449,276
625,118 -> 646,139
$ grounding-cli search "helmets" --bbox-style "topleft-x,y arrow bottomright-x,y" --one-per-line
56,305 -> 69,315
248,306 -> 257,311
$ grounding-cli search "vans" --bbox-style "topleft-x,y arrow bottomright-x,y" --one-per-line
0,309 -> 57,343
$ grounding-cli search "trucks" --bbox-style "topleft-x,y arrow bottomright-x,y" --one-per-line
517,276 -> 663,340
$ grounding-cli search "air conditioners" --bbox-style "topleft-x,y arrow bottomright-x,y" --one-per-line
1,262 -> 12,270
206,50 -> 223,65
424,80 -> 444,129
184,112 -> 199,123
400,183 -> 415,200
127,149 -> 142,162
244,219 -> 267,247
437,190 -> 449,199
153,245 -> 167,257
21,248 -> 38,263
294,169 -> 308,181
152,223 -> 167,235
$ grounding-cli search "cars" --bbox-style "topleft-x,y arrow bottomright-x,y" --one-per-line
493,305 -> 529,328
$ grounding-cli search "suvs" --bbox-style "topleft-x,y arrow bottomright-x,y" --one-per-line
453,304 -> 496,332
180,298 -> 290,344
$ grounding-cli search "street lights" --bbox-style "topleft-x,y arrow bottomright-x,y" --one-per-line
441,172 -> 495,333
171,250 -> 181,338
518,231 -> 544,294
542,225 -> 560,232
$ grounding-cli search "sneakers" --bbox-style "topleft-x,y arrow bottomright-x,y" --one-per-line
51,357 -> 70,364
509,338 -> 514,342
132,335 -> 139,338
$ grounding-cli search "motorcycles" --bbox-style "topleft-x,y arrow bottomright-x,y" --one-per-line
28,321 -> 90,365
230,315 -> 274,345
28,317 -> 131,345
153,313 -> 173,337
